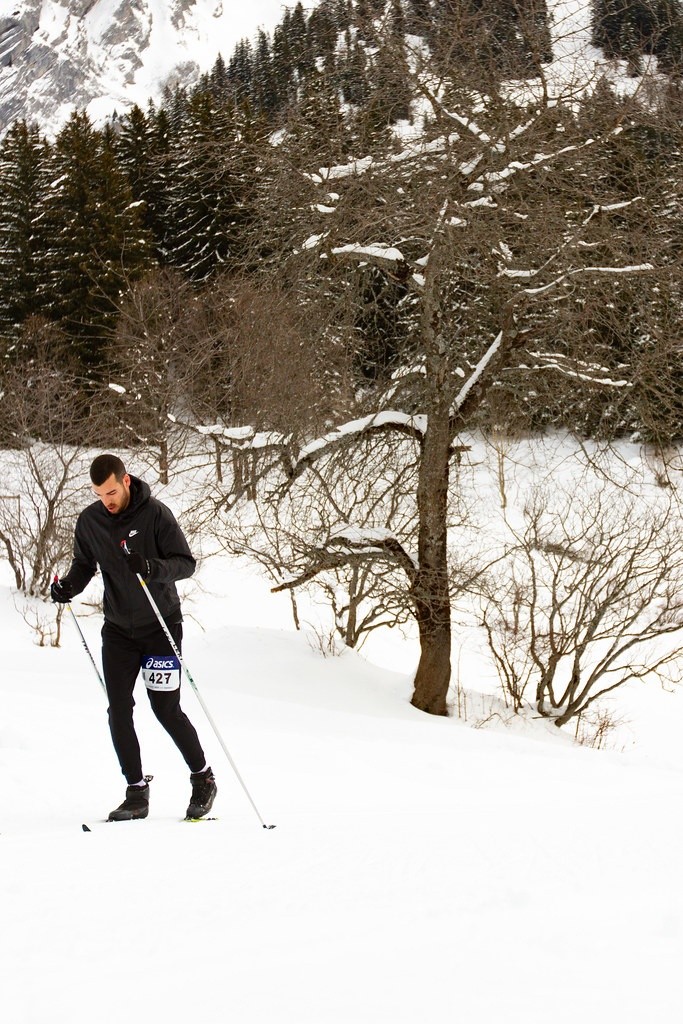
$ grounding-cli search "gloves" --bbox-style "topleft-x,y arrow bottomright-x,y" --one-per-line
51,580 -> 75,604
125,549 -> 150,578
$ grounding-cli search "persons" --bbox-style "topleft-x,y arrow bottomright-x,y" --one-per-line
48,455 -> 217,823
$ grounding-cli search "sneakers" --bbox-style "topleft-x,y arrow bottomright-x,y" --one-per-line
186,767 -> 217,819
109,775 -> 153,821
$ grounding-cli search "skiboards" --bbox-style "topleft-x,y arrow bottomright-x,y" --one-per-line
81,817 -> 223,830
